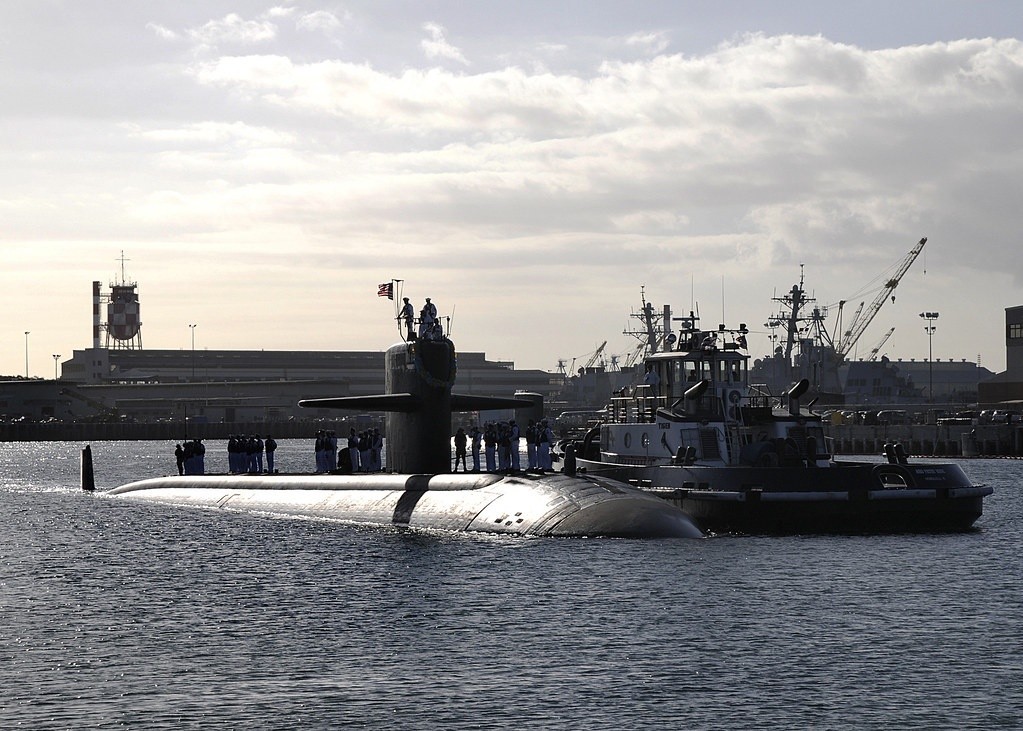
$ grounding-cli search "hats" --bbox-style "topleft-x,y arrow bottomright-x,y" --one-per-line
403,298 -> 409,300
426,298 -> 431,300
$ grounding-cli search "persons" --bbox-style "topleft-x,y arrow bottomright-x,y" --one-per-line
227,432 -> 264,473
686,369 -> 697,380
174,436 -> 205,476
315,427 -> 338,474
453,419 -> 553,471
644,362 -> 661,422
265,434 -> 277,473
419,298 -> 443,340
398,298 -> 414,333
347,425 -> 384,472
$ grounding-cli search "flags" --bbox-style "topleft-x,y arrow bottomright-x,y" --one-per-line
377,283 -> 393,300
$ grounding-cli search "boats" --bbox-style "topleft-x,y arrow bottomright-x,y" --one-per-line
535,275 -> 994,534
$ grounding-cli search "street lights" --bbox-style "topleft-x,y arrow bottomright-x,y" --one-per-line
189,325 -> 196,382
25,332 -> 30,378
53,355 -> 60,421
920,312 -> 939,400
